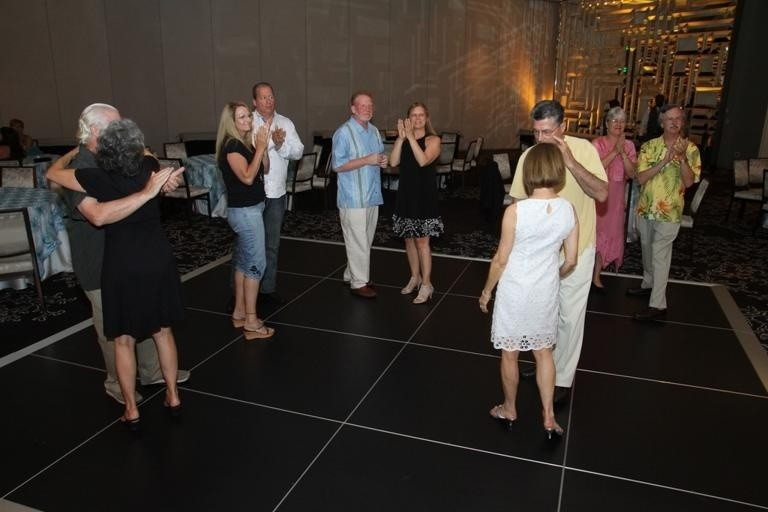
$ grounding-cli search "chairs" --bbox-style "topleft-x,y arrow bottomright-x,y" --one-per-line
452,141 -> 475,194
155,156 -> 212,226
164,141 -> 187,159
284,152 -> 319,218
497,193 -> 513,204
725,160 -> 762,223
313,152 -> 332,211
433,134 -> 458,195
760,169 -> 767,229
0,166 -> 37,191
680,178 -> 710,261
310,143 -> 324,172
493,152 -> 513,190
473,138 -> 484,162
0,207 -> 45,314
0,159 -> 20,166
748,158 -> 768,192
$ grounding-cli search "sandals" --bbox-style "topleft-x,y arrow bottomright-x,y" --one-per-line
232,316 -> 264,330
242,319 -> 275,341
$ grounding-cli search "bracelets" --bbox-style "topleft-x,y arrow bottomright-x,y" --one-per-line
622,157 -> 629,160
482,290 -> 491,299
680,158 -> 689,164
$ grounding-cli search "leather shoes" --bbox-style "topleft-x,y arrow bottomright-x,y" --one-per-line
626,287 -> 652,294
521,367 -> 536,380
632,307 -> 668,321
352,285 -> 376,298
591,281 -> 610,295
552,388 -> 568,407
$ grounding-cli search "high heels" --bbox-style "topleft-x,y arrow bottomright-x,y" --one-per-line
488,404 -> 515,432
544,417 -> 564,442
412,283 -> 434,304
401,275 -> 422,295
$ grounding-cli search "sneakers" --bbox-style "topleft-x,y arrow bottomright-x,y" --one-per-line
105,384 -> 143,405
141,371 -> 191,386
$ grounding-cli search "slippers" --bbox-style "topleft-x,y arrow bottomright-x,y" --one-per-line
117,409 -> 143,432
160,390 -> 184,417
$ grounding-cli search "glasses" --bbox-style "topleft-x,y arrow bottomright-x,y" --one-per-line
531,124 -> 560,136
607,119 -> 626,123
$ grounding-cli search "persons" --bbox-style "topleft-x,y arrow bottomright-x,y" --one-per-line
214,100 -> 276,341
10,119 -> 33,150
225,82 -> 304,317
601,98 -> 620,137
647,95 -> 665,138
683,134 -> 710,199
509,100 -> 609,408
590,107 -> 638,296
390,102 -> 441,306
45,118 -> 184,432
331,91 -> 388,298
49,103 -> 191,406
0,127 -> 25,161
479,142 -> 579,446
626,103 -> 702,321
